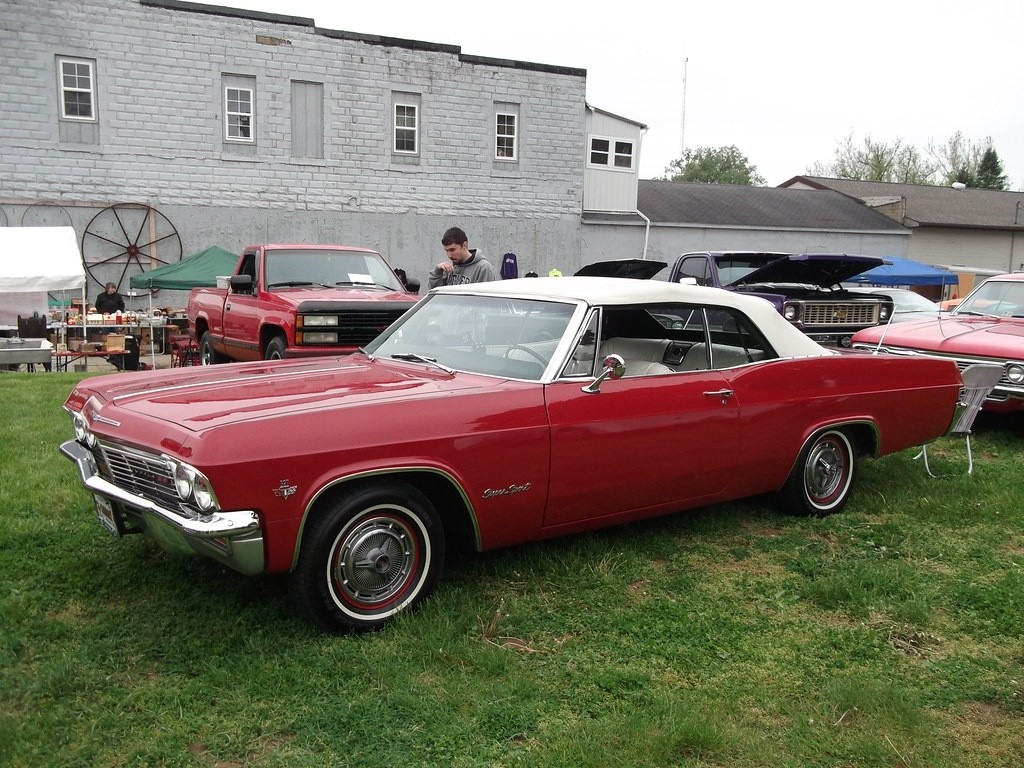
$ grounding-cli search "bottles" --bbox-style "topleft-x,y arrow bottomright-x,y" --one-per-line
116,309 -> 122,325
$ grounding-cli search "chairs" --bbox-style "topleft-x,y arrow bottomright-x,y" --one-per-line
911,364 -> 1005,478
169,334 -> 200,369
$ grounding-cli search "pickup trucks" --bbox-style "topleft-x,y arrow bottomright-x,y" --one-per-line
186,243 -> 422,362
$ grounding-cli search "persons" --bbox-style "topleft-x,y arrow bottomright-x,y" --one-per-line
95,282 -> 125,334
427,227 -> 495,345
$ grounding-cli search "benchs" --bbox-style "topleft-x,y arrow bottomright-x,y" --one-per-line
599,338 -> 765,373
501,349 -> 675,378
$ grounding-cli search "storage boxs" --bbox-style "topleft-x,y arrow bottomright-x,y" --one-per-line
80,344 -> 95,353
103,315 -> 116,325
216,275 -> 232,290
87,314 -> 103,325
69,341 -> 84,350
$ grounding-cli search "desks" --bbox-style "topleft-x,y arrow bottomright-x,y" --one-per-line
0,318 -> 189,373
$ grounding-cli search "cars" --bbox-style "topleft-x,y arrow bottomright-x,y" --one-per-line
849,273 -> 1024,412
57,276 -> 967,638
519,249 -> 1017,350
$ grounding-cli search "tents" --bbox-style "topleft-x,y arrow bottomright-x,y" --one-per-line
847,256 -> 959,299
130,245 -> 240,370
0,226 -> 87,371
